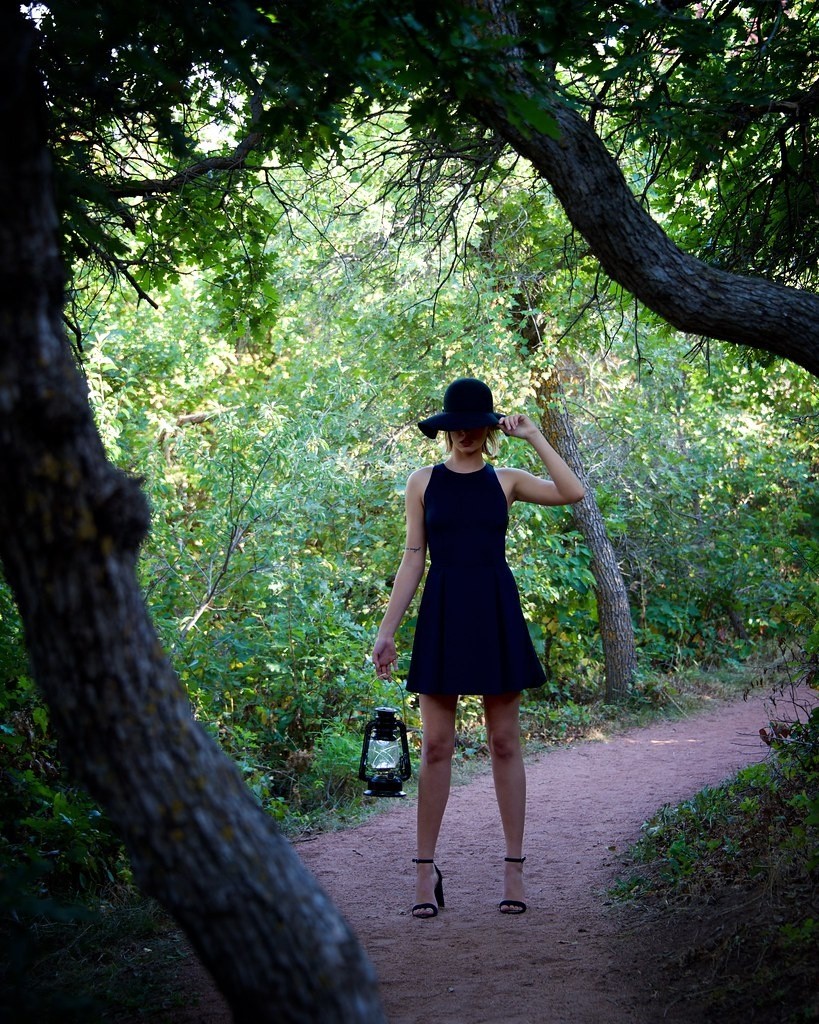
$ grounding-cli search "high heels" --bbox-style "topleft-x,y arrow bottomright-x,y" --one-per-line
411,858 -> 444,917
499,857 -> 526,914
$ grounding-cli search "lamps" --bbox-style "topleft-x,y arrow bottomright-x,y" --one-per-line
360,673 -> 411,797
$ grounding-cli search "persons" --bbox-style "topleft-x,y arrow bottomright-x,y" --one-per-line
371,378 -> 586,919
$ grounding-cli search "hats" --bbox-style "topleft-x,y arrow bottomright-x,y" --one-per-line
417,378 -> 507,440
